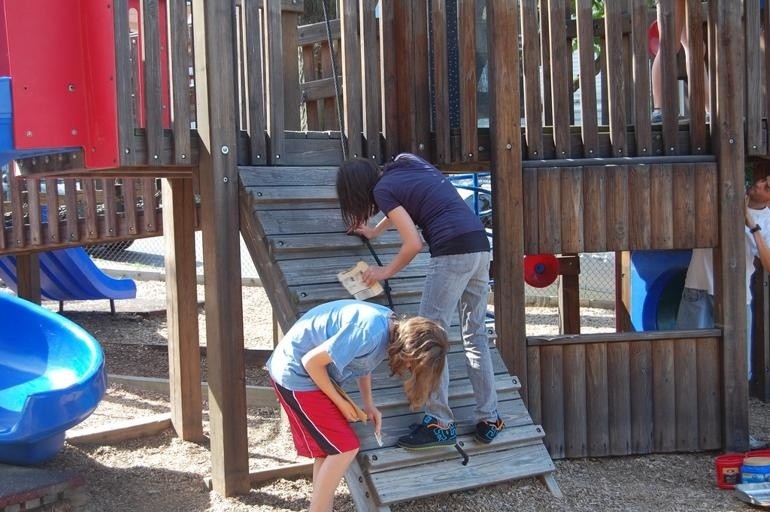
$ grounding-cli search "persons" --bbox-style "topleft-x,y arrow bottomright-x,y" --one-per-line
651,0 -> 712,125
266,298 -> 449,511
675,175 -> 770,450
335,153 -> 505,452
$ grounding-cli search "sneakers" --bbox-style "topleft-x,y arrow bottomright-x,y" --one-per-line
474,417 -> 506,445
397,415 -> 457,450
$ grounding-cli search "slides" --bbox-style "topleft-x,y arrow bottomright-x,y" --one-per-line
0,0 -> 138,466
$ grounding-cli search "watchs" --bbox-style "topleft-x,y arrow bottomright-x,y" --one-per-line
750,224 -> 762,234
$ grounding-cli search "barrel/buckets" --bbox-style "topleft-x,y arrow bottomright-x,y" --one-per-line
745,450 -> 770,457
741,457 -> 770,484
714,453 -> 744,489
